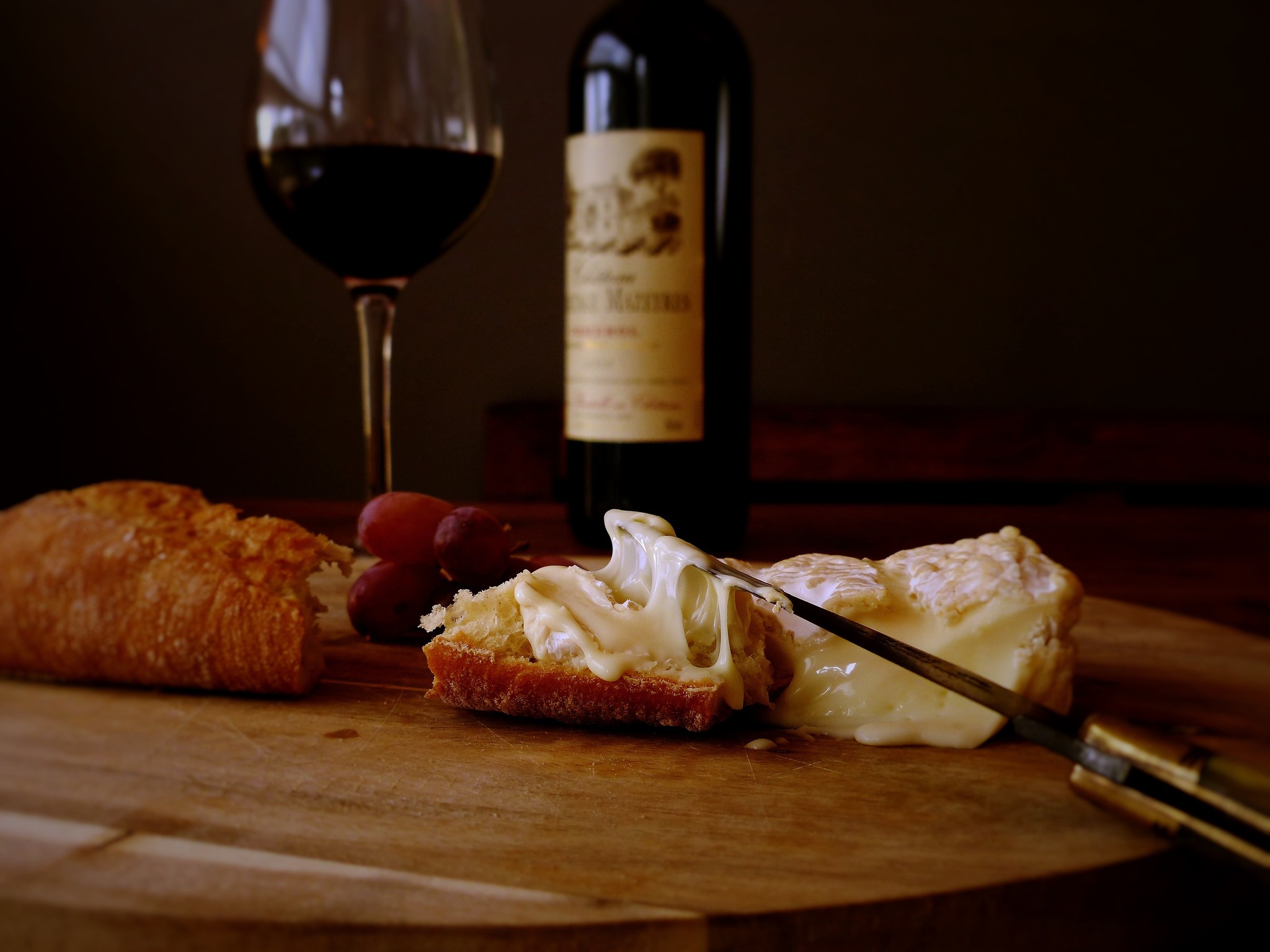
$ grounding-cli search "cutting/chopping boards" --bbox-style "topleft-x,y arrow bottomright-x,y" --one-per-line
0,586 -> 1268,951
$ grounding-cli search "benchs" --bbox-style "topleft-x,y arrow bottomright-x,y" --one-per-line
483,409 -> 1270,638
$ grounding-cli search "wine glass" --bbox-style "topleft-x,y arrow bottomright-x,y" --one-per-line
245,0 -> 502,502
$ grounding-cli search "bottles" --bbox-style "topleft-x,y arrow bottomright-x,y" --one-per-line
565,1 -> 756,559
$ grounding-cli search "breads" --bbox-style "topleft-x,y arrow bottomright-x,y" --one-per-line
0,477 -> 355,698
425,574 -> 791,730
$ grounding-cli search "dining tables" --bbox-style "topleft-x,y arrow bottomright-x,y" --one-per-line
0,556 -> 1270,952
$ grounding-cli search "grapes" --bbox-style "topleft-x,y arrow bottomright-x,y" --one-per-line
345,491 -> 577,639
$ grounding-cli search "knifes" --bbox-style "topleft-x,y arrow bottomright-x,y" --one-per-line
700,548 -> 1269,876
